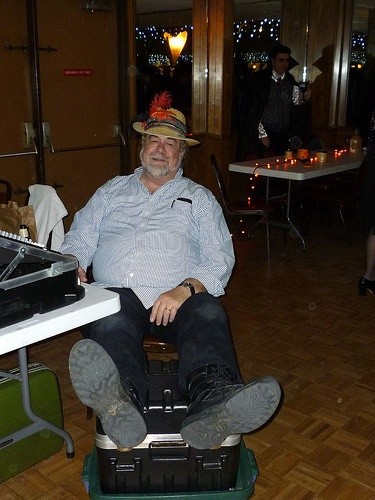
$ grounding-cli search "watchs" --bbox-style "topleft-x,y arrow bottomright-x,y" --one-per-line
178,283 -> 195,295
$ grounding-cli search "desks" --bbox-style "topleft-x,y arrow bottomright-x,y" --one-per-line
0,283 -> 122,459
229,149 -> 367,252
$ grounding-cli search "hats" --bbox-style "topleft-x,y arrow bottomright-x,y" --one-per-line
132,92 -> 198,147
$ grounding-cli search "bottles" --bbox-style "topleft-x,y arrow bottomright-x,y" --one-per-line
349,130 -> 361,153
285,138 -> 292,160
19,225 -> 30,240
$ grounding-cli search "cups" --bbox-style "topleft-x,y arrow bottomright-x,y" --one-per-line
297,149 -> 308,160
316,152 -> 327,162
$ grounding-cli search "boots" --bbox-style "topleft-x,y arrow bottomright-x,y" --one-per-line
179,366 -> 280,449
68,339 -> 148,449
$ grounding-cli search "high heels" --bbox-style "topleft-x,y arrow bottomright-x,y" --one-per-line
358,277 -> 375,295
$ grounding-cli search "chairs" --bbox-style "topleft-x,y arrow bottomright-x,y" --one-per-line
211,155 -> 278,238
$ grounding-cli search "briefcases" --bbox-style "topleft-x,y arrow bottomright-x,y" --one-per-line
0,362 -> 65,483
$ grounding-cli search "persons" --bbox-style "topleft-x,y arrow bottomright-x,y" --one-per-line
56,107 -> 282,449
249,44 -> 303,148
358,111 -> 375,297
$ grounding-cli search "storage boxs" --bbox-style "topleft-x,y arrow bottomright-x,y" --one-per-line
83,386 -> 260,500
0,231 -> 84,328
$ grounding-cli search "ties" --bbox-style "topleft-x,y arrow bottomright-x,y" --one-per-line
277,77 -> 281,82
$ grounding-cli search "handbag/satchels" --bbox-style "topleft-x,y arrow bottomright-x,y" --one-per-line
0,201 -> 37,243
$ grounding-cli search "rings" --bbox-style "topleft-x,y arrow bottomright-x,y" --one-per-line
166,308 -> 170,311
